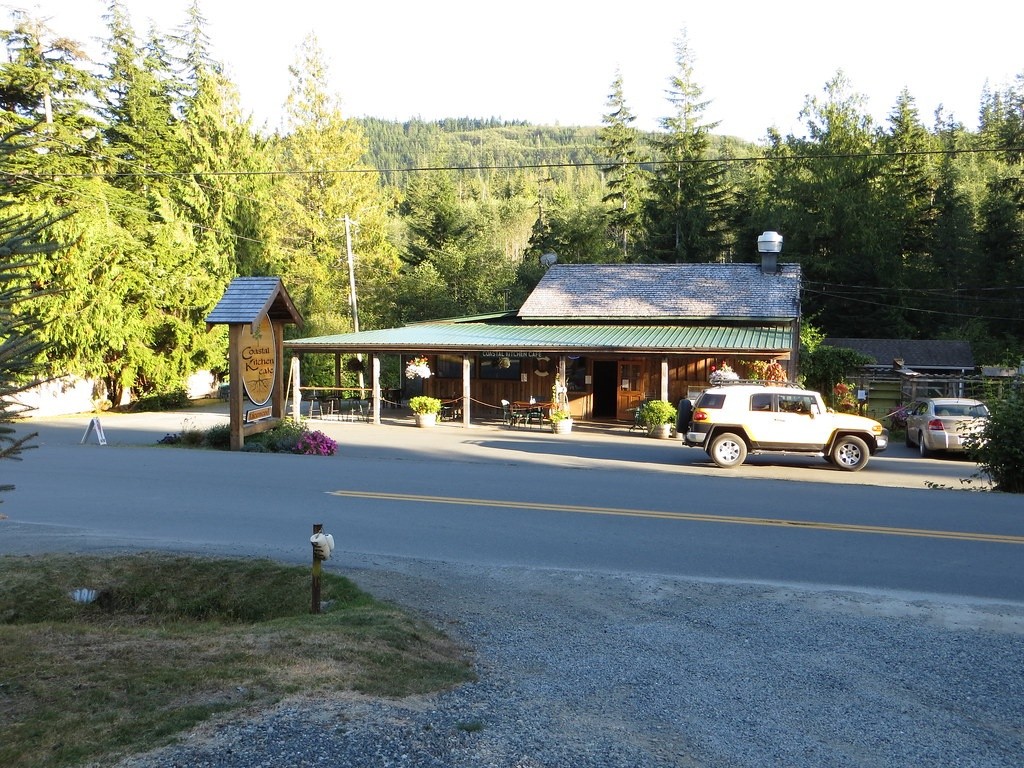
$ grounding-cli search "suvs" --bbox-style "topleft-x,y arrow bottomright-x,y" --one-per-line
676,380 -> 890,471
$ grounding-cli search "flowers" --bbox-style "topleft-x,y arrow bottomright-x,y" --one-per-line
708,365 -> 740,387
739,358 -> 788,387
405,355 -> 431,380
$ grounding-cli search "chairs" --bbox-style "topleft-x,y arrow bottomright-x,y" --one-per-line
500,399 -> 522,426
307,386 -> 324,421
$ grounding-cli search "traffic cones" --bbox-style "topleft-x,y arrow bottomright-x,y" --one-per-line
81,416 -> 107,446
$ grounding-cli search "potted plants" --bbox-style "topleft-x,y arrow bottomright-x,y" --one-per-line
642,399 -> 678,439
407,396 -> 443,428
549,408 -> 574,435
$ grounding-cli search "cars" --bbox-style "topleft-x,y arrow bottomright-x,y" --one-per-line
906,397 -> 995,457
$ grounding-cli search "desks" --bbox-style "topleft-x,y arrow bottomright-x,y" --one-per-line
382,388 -> 464,424
513,400 -> 553,429
625,407 -> 646,433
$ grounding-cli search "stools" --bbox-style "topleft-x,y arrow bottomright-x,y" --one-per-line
364,388 -> 382,424
326,386 -> 345,422
345,386 -> 364,423
